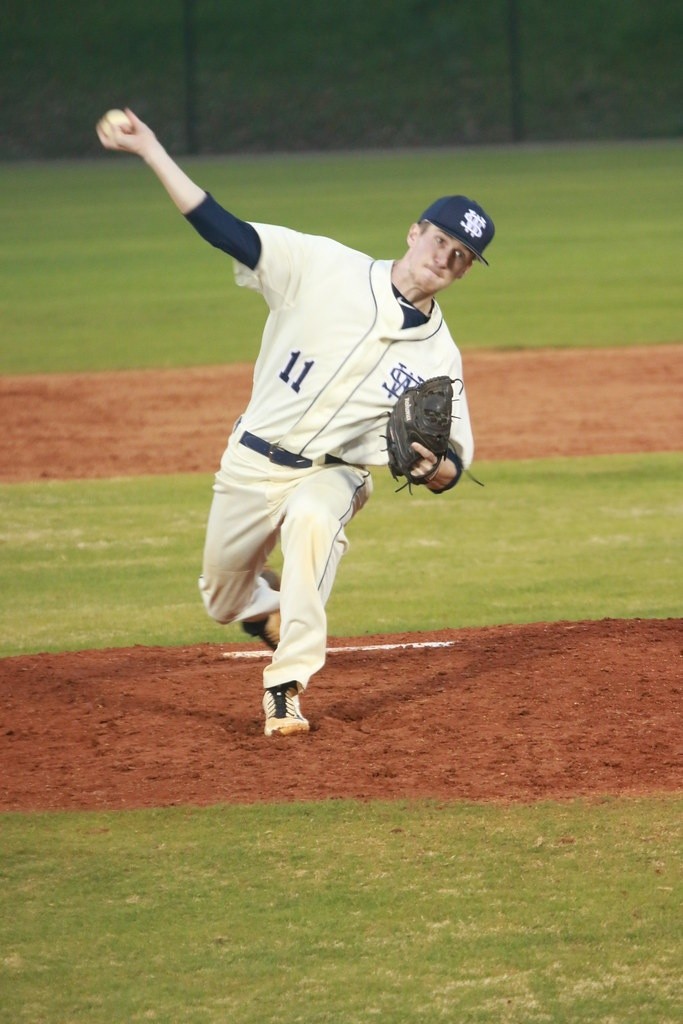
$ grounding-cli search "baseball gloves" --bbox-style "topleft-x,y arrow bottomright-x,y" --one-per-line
387,375 -> 454,488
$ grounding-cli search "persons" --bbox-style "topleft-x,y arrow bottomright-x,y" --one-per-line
97,106 -> 496,735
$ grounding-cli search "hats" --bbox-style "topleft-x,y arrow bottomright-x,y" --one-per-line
418,195 -> 495,266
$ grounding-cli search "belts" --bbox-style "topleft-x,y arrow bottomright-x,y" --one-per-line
232,417 -> 347,469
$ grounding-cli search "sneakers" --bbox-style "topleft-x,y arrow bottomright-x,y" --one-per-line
244,570 -> 281,651
262,683 -> 310,736
